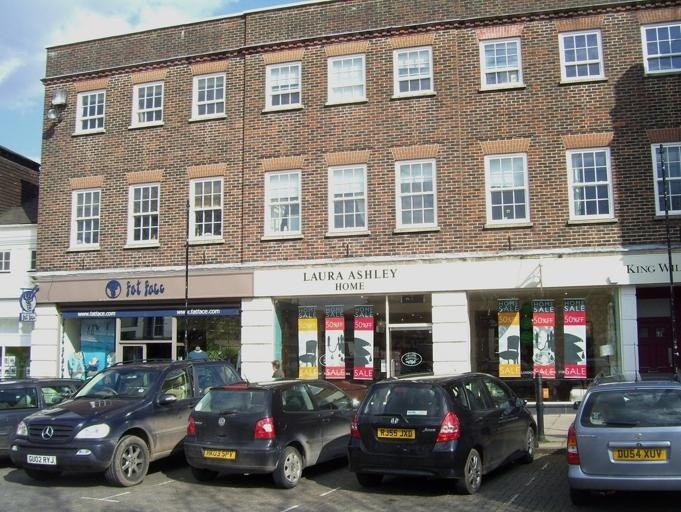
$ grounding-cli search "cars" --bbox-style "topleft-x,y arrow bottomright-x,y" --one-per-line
184,378 -> 362,489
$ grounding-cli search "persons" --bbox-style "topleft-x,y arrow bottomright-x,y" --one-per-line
68,351 -> 86,381
271,360 -> 285,377
187,347 -> 210,386
104,352 -> 115,384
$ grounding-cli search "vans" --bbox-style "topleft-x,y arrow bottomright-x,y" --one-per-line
1,377 -> 87,460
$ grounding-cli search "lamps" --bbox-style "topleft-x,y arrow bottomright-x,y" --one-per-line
46,88 -> 68,122
600,343 -> 617,375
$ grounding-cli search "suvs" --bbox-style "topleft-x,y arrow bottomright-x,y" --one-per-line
347,370 -> 542,495
565,369 -> 681,500
10,357 -> 248,491
